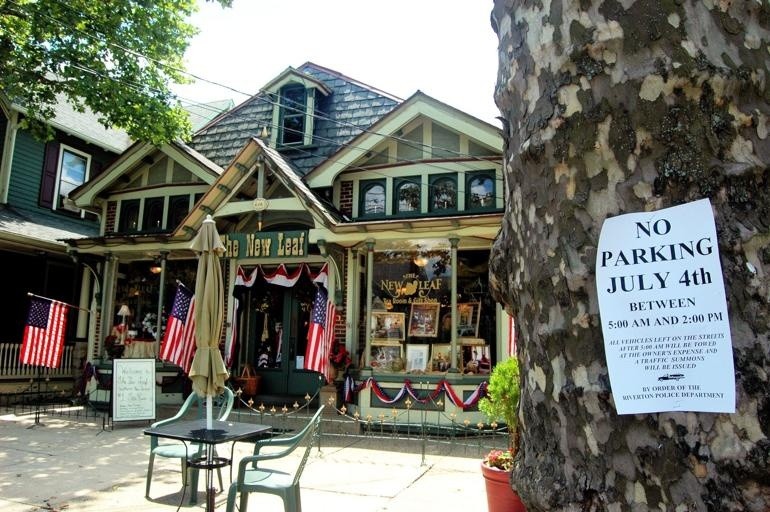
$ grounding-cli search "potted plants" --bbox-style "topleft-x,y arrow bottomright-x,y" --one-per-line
477,356 -> 526,512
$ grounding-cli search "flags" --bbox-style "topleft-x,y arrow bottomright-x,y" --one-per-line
304,290 -> 337,383
159,283 -> 198,374
17,295 -> 69,369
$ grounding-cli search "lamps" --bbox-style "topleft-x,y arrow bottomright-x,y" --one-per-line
116,304 -> 131,325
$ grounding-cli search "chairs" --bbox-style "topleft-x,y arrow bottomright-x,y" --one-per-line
144,385 -> 326,512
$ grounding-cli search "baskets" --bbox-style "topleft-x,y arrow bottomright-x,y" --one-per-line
235,366 -> 261,396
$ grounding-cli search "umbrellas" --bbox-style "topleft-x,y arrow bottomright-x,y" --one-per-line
186,212 -> 229,511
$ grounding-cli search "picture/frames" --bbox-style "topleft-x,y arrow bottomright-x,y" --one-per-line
369,302 -> 491,375
